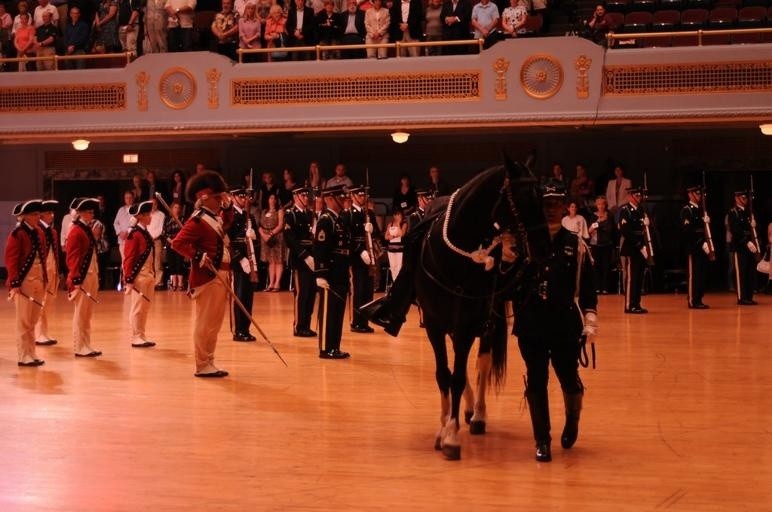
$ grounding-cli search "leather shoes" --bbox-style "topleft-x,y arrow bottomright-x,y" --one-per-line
738,299 -> 757,304
75,352 -> 101,357
689,303 -> 709,308
36,340 -> 57,345
233,333 -> 257,341
624,307 -> 648,313
351,326 -> 374,333
133,342 -> 155,347
196,371 -> 228,377
18,359 -> 44,366
294,328 -> 317,337
320,349 -> 350,358
272,288 -> 280,292
264,288 -> 273,292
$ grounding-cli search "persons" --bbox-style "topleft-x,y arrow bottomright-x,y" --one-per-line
531,159 -> 772,314
0,158 -> 453,358
5,200 -> 46,366
170,170 -> 235,377
2,2 -> 633,74
506,182 -> 598,462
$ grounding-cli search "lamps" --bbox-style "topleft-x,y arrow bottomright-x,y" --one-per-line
72,137 -> 91,152
757,122 -> 772,136
390,130 -> 411,145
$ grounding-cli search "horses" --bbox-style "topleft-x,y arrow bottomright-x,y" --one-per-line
407,153 -> 554,462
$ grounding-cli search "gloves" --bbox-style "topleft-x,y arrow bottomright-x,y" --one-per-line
500,231 -> 518,263
641,246 -> 648,259
304,255 -> 315,272
702,241 -> 710,255
239,257 -> 251,274
747,241 -> 758,253
644,217 -> 650,226
364,223 -> 373,233
360,250 -> 371,265
581,313 -> 597,339
316,277 -> 330,290
702,215 -> 711,223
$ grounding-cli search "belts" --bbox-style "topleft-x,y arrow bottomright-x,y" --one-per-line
296,240 -> 313,245
355,237 -> 365,242
633,231 -> 642,235
334,249 -> 350,255
232,237 -> 246,242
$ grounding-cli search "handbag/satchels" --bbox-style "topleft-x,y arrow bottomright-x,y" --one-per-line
269,33 -> 288,58
757,251 -> 771,274
266,232 -> 278,248
93,30 -> 105,53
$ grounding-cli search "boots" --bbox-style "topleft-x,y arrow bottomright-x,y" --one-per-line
561,387 -> 583,447
526,390 -> 551,461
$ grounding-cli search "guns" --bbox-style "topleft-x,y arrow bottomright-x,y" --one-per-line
701,168 -> 716,261
365,167 -> 377,276
246,167 -> 259,283
749,173 -> 762,259
642,171 -> 656,265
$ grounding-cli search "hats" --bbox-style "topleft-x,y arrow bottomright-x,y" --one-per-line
347,184 -> 365,193
686,184 -> 703,191
12,199 -> 42,217
228,185 -> 246,195
625,185 -> 641,194
538,178 -> 567,199
41,199 -> 60,212
129,200 -> 153,216
415,188 -> 433,194
290,185 -> 310,194
321,183 -> 346,196
184,170 -> 228,203
68,197 -> 100,209
734,189 -> 747,195
